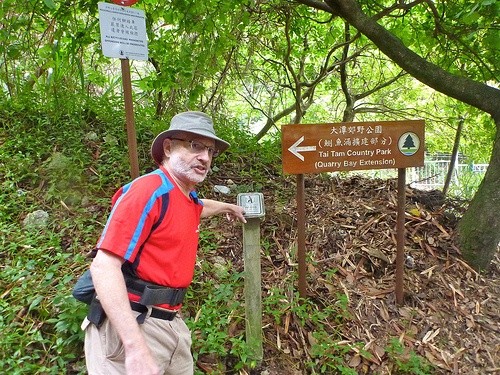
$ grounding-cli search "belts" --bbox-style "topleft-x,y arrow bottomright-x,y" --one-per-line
129,301 -> 177,321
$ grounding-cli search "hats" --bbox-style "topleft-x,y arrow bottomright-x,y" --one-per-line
151,111 -> 231,163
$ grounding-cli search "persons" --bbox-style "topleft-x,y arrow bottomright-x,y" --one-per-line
72,111 -> 247,375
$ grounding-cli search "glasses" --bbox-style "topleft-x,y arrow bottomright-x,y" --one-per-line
169,137 -> 220,158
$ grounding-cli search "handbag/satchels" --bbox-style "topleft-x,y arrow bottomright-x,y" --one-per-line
73,271 -> 96,305
